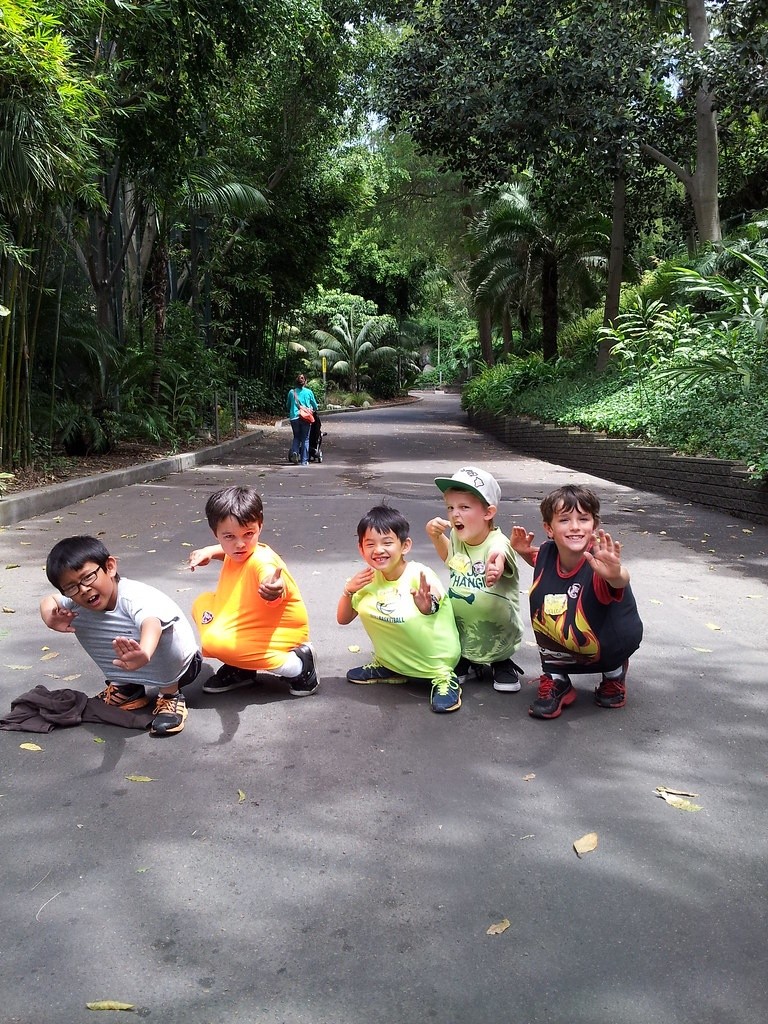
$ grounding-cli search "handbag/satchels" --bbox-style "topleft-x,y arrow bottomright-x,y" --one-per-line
299,405 -> 316,423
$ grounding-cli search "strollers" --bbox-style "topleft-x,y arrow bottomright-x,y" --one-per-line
288,411 -> 327,464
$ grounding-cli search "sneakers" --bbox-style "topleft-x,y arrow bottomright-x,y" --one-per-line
289,643 -> 321,696
150,694 -> 188,734
595,658 -> 629,707
432,677 -> 463,712
93,683 -> 149,710
529,674 -> 577,718
202,663 -> 257,693
491,659 -> 521,692
454,658 -> 486,684
347,663 -> 408,684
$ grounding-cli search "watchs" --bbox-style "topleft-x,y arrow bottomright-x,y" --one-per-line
429,593 -> 440,613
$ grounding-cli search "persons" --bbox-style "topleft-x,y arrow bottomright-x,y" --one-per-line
189,484 -> 320,697
336,496 -> 462,714
510,485 -> 643,718
287,375 -> 318,465
425,467 -> 525,692
39,536 -> 204,735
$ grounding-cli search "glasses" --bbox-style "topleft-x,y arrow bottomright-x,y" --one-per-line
61,566 -> 100,598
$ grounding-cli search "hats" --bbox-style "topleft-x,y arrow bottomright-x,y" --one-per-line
434,466 -> 501,508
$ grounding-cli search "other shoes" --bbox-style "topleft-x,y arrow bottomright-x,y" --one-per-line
293,452 -> 299,464
